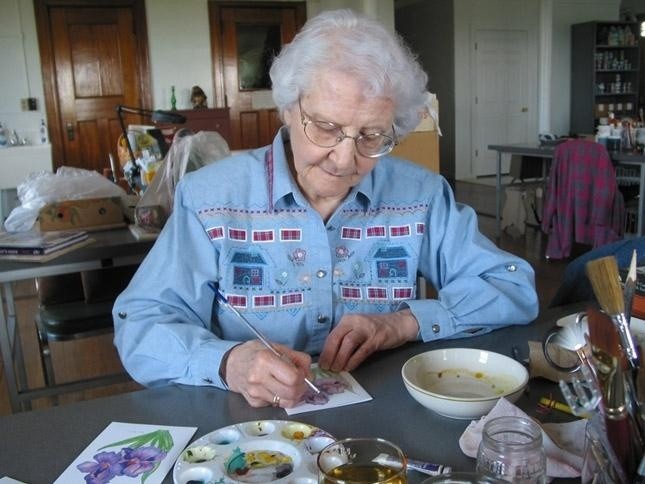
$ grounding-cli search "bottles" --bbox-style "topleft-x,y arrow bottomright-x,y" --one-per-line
103,169 -> 114,182
420,471 -> 512,484
608,112 -> 617,128
9,130 -> 18,145
596,117 -> 610,149
171,86 -> 176,109
39,119 -> 48,144
0,124 -> 7,145
595,26 -> 638,117
476,415 -> 548,484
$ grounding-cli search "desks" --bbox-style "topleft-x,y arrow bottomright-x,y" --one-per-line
2,303 -> 645,484
1,187 -> 156,413
488,143 -> 645,239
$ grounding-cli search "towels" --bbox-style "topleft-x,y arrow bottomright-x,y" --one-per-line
459,398 -> 599,484
501,187 -> 527,235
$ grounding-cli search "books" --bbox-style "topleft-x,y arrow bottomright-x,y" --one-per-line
128,223 -> 160,241
0,229 -> 97,264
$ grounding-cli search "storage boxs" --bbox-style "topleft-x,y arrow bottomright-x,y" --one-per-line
412,94 -> 442,132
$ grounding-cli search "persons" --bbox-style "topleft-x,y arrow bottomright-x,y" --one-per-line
111,7 -> 540,409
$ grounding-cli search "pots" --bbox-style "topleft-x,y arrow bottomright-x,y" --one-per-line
41,300 -> 117,338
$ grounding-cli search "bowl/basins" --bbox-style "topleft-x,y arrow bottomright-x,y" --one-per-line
401,346 -> 530,420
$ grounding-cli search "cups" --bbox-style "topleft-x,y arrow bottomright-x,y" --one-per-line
316,436 -> 406,484
605,136 -> 621,151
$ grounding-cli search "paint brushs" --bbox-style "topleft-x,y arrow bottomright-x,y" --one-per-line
557,256 -> 642,483
207,281 -> 330,403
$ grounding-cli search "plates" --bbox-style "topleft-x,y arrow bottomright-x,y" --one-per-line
557,309 -> 645,349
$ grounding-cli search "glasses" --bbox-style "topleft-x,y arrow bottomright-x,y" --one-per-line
298,97 -> 401,159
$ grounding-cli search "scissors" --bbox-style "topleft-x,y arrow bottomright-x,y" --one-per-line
544,311 -> 590,374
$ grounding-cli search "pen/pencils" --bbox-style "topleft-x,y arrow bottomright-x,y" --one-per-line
624,247 -> 637,323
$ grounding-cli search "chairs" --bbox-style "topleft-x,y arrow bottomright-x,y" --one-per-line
34,262 -> 141,406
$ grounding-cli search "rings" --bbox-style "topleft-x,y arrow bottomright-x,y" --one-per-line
272,395 -> 281,409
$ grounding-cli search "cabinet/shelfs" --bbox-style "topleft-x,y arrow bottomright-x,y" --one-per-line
151,107 -> 232,150
570,21 -> 645,138
